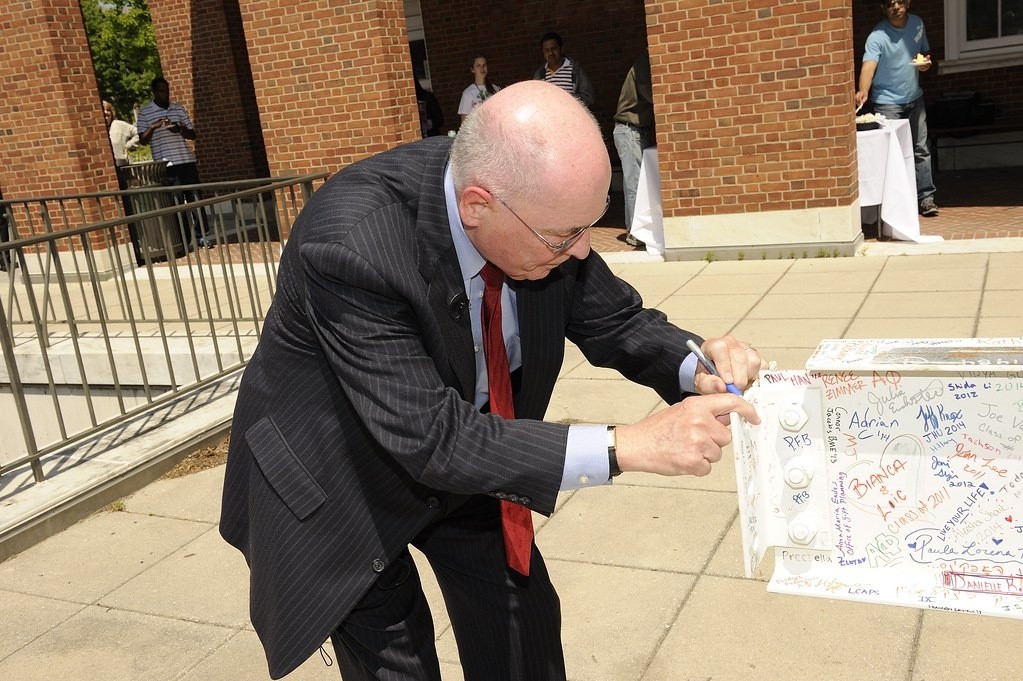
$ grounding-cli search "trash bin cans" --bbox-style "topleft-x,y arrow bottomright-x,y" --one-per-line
119,159 -> 193,259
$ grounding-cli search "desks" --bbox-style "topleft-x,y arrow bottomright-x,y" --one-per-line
631,119 -> 913,242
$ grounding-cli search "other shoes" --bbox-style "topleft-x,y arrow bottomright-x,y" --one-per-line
196,234 -> 211,249
625,232 -> 646,246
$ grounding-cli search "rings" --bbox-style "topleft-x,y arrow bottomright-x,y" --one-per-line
745,348 -> 757,352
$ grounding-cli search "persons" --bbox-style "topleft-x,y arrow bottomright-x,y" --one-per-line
102,100 -> 139,198
855,0 -> 940,216
613,45 -> 653,245
458,51 -> 502,123
413,72 -> 445,138
220,80 -> 769,681
533,32 -> 595,106
137,78 -> 213,252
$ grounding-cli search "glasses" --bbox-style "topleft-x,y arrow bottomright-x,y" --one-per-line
885,0 -> 907,8
482,186 -> 610,254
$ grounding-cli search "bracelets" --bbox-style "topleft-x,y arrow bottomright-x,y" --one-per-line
180,127 -> 184,135
606,425 -> 622,476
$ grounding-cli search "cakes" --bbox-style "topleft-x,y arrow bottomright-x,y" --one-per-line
912,53 -> 927,63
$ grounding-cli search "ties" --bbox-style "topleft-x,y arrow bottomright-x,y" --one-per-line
478,261 -> 534,577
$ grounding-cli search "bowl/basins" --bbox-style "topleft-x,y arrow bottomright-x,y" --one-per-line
857,122 -> 880,131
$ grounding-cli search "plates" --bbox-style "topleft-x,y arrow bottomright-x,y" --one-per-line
910,61 -> 931,66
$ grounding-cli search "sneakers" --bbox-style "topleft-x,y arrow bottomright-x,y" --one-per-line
921,196 -> 939,214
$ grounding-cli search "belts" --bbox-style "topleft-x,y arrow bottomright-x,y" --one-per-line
615,120 -> 637,127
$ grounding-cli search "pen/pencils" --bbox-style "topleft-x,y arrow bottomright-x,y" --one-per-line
687,338 -> 746,396
